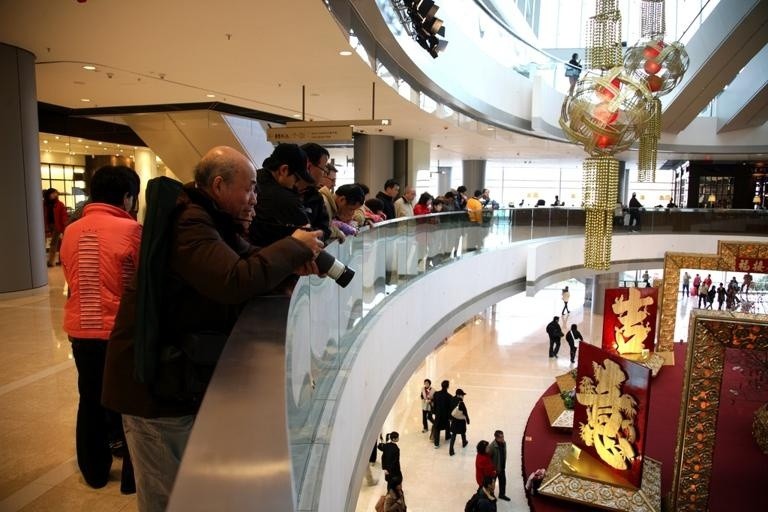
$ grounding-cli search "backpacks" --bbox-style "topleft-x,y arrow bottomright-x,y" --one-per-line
574,339 -> 581,347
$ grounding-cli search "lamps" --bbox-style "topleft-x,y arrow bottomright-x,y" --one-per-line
752,196 -> 761,209
708,193 -> 716,208
402,0 -> 446,58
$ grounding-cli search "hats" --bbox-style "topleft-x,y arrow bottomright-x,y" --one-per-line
455,389 -> 467,395
272,143 -> 316,184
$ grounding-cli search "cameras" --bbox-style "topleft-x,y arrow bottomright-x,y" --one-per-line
317,250 -> 356,289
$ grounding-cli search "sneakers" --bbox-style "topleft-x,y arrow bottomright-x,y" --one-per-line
421,427 -> 469,456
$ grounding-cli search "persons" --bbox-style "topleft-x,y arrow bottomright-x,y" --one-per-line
646,283 -> 651,288
741,273 -> 752,292
245,143 -> 311,248
300,142 -> 332,248
384,475 -> 407,512
465,476 -> 497,512
318,184 -> 364,244
693,274 -> 702,296
102,145 -> 325,512
431,380 -> 455,449
490,430 -> 510,501
717,282 -> 729,310
318,166 -> 328,187
698,281 -> 709,308
554,195 -> 560,206
708,285 -> 716,310
642,270 -> 649,282
726,277 -> 741,311
682,272 -> 691,297
566,324 -> 583,363
569,53 -> 582,97
475,440 -> 497,486
352,179 -> 499,315
325,163 -> 337,190
562,286 -> 570,315
420,379 -> 436,433
377,431 -> 400,493
628,192 -> 643,233
449,389 -> 470,456
546,316 -> 564,358
667,198 -> 678,208
241,189 -> 257,233
704,274 -> 712,289
43,188 -> 69,267
364,439 -> 379,485
59,166 -> 135,494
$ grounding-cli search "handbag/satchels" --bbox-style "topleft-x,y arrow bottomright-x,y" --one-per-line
131,176 -> 186,386
375,495 -> 396,512
624,214 -> 636,226
451,407 -> 466,420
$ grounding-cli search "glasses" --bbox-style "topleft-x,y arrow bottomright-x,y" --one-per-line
317,165 -> 329,173
